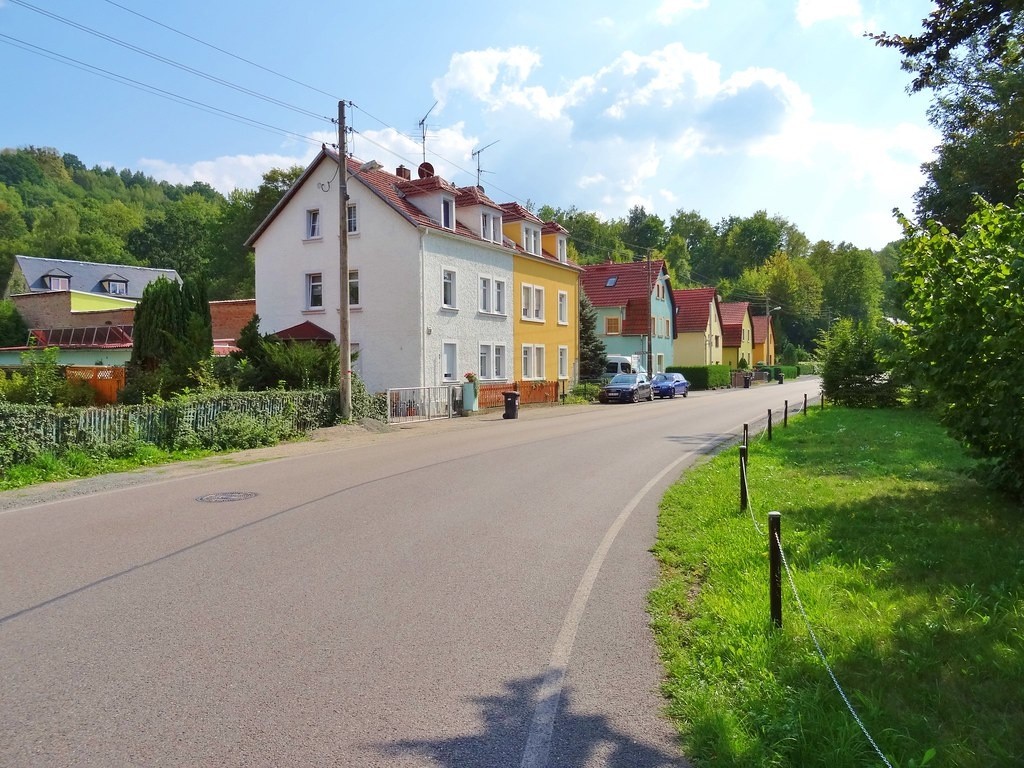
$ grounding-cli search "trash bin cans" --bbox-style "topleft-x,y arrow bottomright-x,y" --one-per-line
743,376 -> 750,388
501,390 -> 521,420
779,373 -> 785,384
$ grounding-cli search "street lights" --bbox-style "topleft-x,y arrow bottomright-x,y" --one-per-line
766,306 -> 783,365
827,317 -> 841,335
338,159 -> 385,422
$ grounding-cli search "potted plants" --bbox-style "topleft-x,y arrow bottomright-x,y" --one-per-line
406,400 -> 418,416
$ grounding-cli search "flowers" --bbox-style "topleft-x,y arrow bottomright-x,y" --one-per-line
464,373 -> 479,398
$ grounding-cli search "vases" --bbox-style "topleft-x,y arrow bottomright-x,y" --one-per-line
468,379 -> 473,382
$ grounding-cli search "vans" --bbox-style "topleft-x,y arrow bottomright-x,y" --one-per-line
603,354 -> 655,378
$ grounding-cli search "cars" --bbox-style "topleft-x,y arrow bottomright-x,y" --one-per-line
650,373 -> 690,399
599,373 -> 654,404
